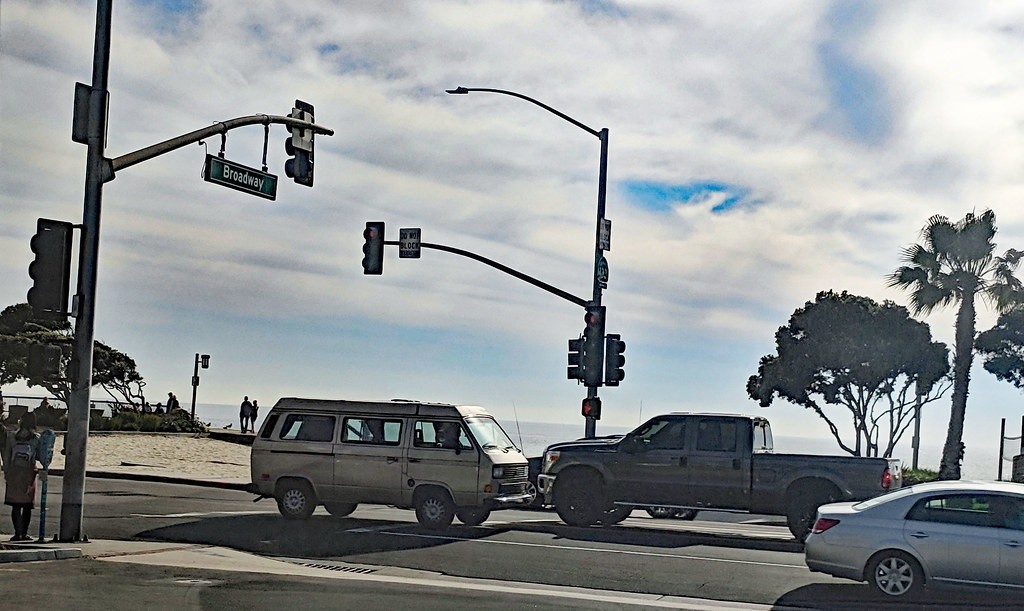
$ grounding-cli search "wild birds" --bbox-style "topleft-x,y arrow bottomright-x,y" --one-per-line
223,423 -> 232,429
206,423 -> 211,427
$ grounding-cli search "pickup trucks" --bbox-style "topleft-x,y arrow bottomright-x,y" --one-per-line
537,412 -> 902,543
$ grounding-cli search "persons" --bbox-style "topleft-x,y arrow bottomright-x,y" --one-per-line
135,402 -> 142,411
154,403 -> 162,413
250,400 -> 259,433
0,412 -> 42,541
166,392 -> 179,413
145,402 -> 152,412
240,396 -> 252,433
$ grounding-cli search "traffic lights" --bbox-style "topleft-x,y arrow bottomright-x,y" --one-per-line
285,100 -> 315,188
362,221 -> 385,275
605,334 -> 626,387
28,218 -> 73,322
567,338 -> 583,380
583,306 -> 606,386
582,398 -> 599,417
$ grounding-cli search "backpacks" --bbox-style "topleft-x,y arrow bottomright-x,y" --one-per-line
10,433 -> 37,467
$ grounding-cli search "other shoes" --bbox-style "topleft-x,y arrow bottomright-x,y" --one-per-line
21,535 -> 34,540
10,534 -> 22,541
241,428 -> 247,433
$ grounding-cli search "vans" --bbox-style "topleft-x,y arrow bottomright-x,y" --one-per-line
246,397 -> 532,530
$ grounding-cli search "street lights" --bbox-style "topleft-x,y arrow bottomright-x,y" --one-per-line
191,353 -> 210,420
445,86 -> 608,439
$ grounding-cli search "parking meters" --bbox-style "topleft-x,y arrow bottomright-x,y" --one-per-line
38,430 -> 55,544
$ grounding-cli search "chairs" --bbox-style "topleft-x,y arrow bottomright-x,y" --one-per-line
415,429 -> 425,443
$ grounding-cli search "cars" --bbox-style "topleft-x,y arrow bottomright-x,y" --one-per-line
804,480 -> 1024,602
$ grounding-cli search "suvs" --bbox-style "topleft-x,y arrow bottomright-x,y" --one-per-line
525,435 -> 699,520
